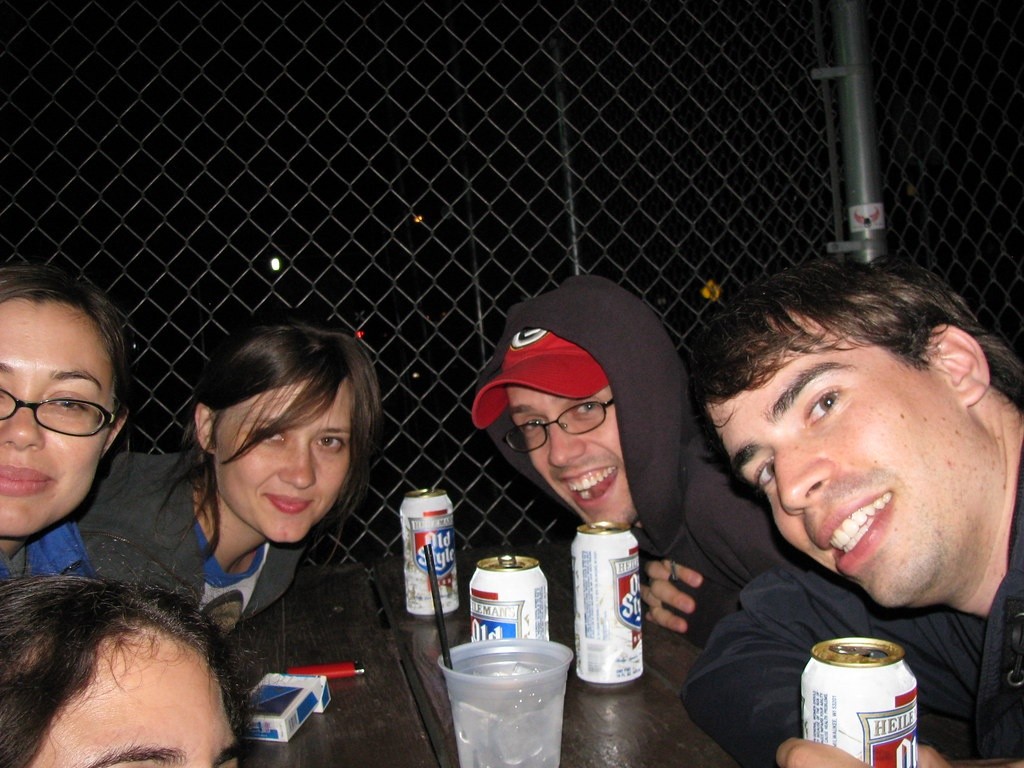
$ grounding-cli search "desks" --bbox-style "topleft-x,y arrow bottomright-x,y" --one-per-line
221,540 -> 979,768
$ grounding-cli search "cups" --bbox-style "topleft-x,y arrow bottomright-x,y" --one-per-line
437,639 -> 573,767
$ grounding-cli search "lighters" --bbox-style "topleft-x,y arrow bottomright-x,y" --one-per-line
287,659 -> 366,678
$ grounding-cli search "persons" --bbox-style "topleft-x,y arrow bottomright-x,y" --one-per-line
470,252 -> 1024,768
0,574 -> 242,768
0,260 -> 382,768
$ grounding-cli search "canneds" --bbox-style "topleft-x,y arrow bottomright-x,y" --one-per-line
468,554 -> 549,640
801,636 -> 917,768
400,488 -> 459,618
570,521 -> 645,686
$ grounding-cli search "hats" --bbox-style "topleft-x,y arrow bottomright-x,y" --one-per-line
471,328 -> 610,430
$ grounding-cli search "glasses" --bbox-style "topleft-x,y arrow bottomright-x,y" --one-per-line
502,398 -> 615,454
0,388 -> 121,437
